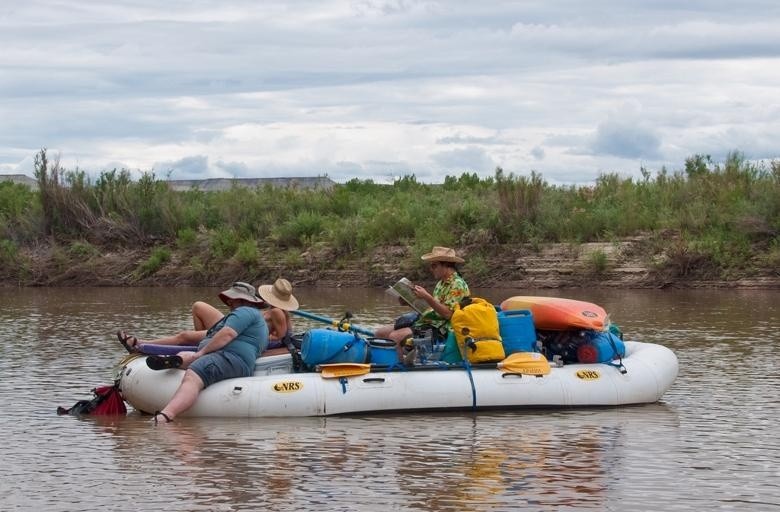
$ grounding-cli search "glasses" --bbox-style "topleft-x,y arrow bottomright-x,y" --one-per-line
432,264 -> 437,269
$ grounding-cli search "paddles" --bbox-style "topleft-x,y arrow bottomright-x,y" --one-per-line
320,352 -> 550,378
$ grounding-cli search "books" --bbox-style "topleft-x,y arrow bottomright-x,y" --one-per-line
385,278 -> 431,316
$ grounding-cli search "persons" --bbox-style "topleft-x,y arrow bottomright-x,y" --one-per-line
146,282 -> 269,424
375,246 -> 471,366
117,277 -> 299,355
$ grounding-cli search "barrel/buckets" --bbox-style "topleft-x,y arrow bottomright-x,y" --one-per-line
497,310 -> 536,357
300,329 -> 368,371
367,338 -> 399,372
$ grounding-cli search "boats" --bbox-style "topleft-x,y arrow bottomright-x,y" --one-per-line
116,339 -> 680,421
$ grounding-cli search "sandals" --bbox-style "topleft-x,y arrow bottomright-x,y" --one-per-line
155,413 -> 173,422
146,354 -> 183,370
117,331 -> 137,354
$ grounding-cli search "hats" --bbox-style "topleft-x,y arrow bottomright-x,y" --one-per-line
218,282 -> 264,306
258,279 -> 299,312
421,246 -> 465,263
